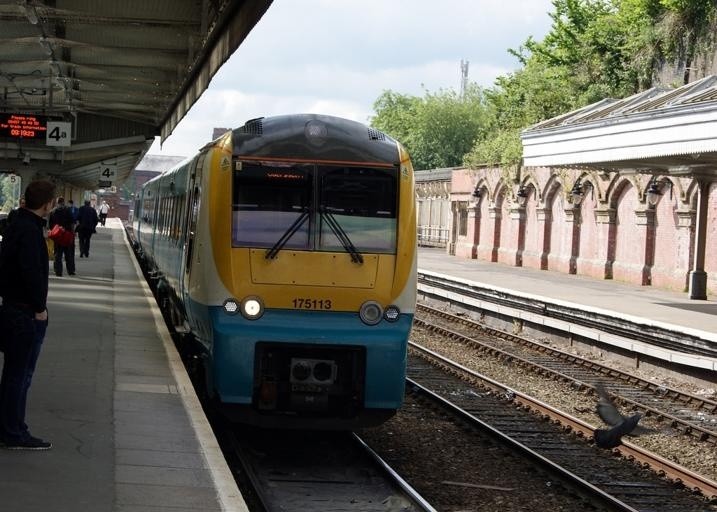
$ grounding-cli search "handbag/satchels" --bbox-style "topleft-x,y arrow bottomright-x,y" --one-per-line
48,223 -> 74,247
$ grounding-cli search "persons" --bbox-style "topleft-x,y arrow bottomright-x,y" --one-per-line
99,201 -> 110,226
75,198 -> 98,261
48,196 -> 77,275
66,200 -> 78,237
0,180 -> 56,451
18,197 -> 26,208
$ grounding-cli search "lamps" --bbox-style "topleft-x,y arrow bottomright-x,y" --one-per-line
572,180 -> 594,205
646,177 -> 673,206
471,187 -> 487,206
19,150 -> 31,165
517,184 -> 536,205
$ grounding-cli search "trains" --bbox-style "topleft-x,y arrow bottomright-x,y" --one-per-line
132,114 -> 419,439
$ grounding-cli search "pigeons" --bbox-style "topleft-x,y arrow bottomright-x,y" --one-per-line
593,378 -> 659,450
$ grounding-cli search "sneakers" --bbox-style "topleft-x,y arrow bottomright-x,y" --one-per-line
55,254 -> 88,277
0,436 -> 53,450
